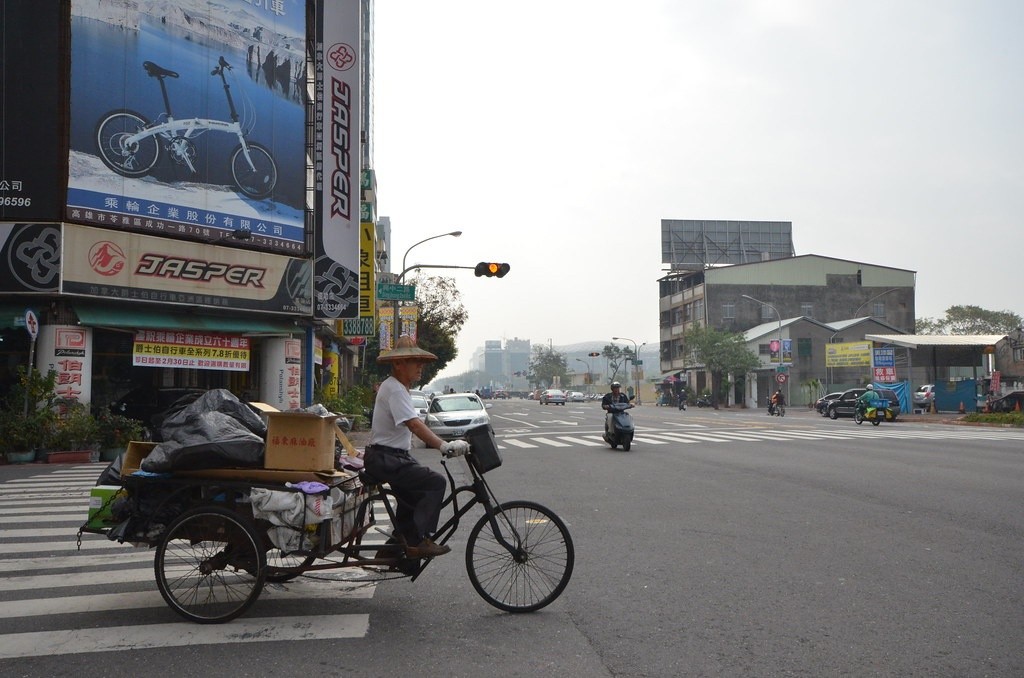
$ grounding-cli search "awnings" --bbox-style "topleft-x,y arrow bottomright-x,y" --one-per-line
651,369 -> 682,382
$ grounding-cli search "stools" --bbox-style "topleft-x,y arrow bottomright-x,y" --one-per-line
913,407 -> 927,414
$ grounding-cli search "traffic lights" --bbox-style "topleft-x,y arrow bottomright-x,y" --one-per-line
475,262 -> 510,278
589,353 -> 600,356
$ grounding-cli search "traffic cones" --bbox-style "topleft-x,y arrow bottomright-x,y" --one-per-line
1015,400 -> 1020,411
960,400 -> 966,414
930,401 -> 937,414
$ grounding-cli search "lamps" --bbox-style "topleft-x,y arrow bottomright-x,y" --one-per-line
210,229 -> 252,245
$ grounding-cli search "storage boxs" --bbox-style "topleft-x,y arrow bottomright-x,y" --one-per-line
87,484 -> 129,529
120,441 -> 163,474
248,401 -> 361,471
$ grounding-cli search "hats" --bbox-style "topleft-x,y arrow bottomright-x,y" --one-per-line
376,334 -> 438,365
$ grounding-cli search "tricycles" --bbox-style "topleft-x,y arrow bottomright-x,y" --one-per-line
77,424 -> 574,625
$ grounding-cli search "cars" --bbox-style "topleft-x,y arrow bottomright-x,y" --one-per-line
914,384 -> 935,408
528,388 -> 606,405
409,389 -> 492,448
493,390 -> 507,399
815,391 -> 845,414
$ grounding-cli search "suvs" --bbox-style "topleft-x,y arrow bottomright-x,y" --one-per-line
827,388 -> 901,419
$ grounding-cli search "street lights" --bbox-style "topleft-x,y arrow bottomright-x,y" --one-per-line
402,231 -> 462,305
613,337 -> 646,404
576,359 -> 590,397
741,295 -> 783,405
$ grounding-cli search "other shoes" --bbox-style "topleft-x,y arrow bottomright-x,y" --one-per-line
375,551 -> 422,573
408,541 -> 451,558
608,432 -> 614,436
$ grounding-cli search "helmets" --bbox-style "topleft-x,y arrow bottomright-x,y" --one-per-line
681,389 -> 685,392
866,384 -> 873,390
776,392 -> 779,395
611,382 -> 621,390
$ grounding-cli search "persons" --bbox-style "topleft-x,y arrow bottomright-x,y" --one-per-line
856,384 -> 879,420
475,390 -> 480,397
364,336 -> 469,574
769,390 -> 784,416
602,382 -> 635,437
450,388 -> 454,393
677,389 -> 688,410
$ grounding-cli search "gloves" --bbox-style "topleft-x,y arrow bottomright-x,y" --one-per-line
439,439 -> 471,457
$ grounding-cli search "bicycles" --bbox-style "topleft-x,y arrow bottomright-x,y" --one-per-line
95,56 -> 279,200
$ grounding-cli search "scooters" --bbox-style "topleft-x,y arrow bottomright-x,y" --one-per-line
678,398 -> 687,410
768,400 -> 785,417
603,395 -> 635,450
696,394 -> 714,407
853,393 -> 885,426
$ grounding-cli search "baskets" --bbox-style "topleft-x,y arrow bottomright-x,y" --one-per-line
465,423 -> 503,474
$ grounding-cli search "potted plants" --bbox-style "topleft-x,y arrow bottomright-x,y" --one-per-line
0,363 -> 144,462
